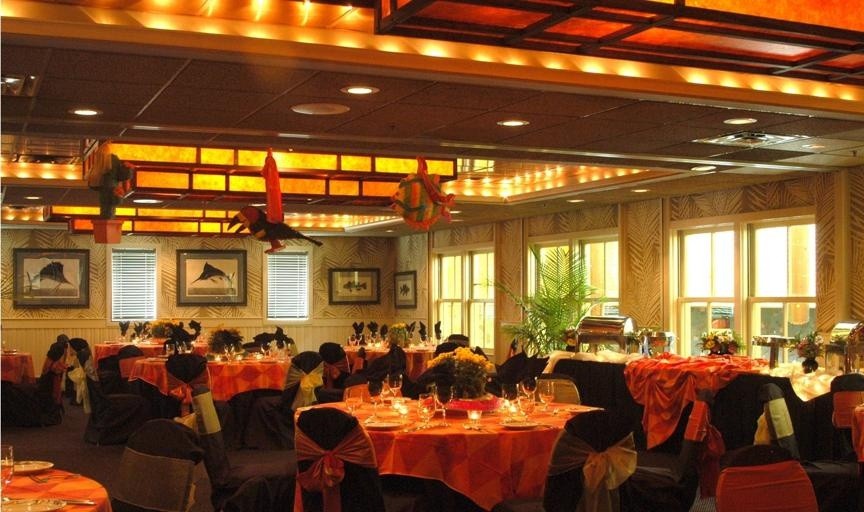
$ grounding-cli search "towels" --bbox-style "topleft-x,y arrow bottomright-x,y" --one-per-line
119,321 -> 130,336
523,351 -> 549,391
495,345 -> 532,399
352,322 -> 364,339
401,367 -> 434,400
133,321 -> 143,337
380,323 -> 388,341
431,360 -> 456,403
274,324 -> 291,349
419,320 -> 427,340
406,322 -> 416,338
435,321 -> 441,340
365,350 -> 391,394
390,343 -> 407,387
367,320 -> 378,338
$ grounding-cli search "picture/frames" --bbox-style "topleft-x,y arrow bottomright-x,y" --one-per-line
328,267 -> 381,305
13,248 -> 89,308
393,271 -> 417,308
176,248 -> 247,307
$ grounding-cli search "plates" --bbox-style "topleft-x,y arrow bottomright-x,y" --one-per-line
13,461 -> 53,475
0,498 -> 67,512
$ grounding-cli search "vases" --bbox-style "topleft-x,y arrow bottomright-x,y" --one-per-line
802,357 -> 818,374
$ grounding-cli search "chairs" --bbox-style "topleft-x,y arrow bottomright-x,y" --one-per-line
716,445 -> 820,512
294,407 -> 434,512
70,338 -> 91,357
165,354 -> 227,429
757,382 -> 860,503
191,385 -> 295,512
12,342 -> 68,427
163,339 -> 193,353
110,418 -> 206,511
253,333 -> 273,344
97,356 -> 154,415
831,374 -> 863,459
77,346 -> 143,446
536,372 -> 583,404
58,334 -> 117,406
632,388 -> 716,512
319,342 -> 351,400
491,410 -> 638,512
232,351 -> 324,448
118,345 -> 143,388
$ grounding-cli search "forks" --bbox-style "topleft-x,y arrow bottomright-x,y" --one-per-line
29,475 -> 80,483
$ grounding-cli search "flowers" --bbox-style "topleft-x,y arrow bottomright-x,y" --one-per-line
208,330 -> 243,352
427,346 -> 493,399
146,320 -> 178,338
695,331 -> 743,354
785,330 -> 824,359
389,323 -> 405,334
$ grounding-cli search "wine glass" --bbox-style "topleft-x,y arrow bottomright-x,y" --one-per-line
0,446 -> 14,504
166,341 -> 291,366
345,374 -> 554,430
349,332 -> 434,351
118,327 -> 151,342
